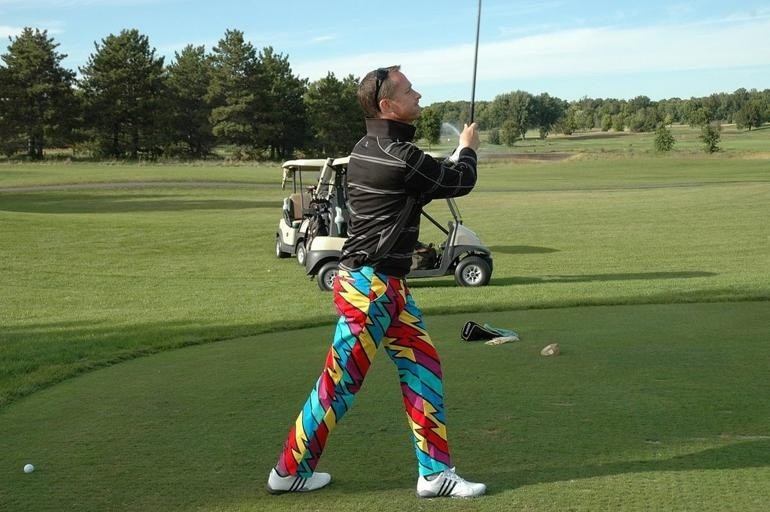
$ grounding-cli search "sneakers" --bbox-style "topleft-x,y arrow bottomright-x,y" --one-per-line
266,465 -> 331,496
416,466 -> 488,499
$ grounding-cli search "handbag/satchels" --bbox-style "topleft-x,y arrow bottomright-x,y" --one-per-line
460,320 -> 501,341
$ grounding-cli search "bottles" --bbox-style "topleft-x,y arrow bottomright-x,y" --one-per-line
540,342 -> 560,357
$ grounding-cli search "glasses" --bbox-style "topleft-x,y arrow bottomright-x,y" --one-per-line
374,66 -> 389,110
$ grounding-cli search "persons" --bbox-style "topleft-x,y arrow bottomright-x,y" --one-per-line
266,65 -> 490,500
